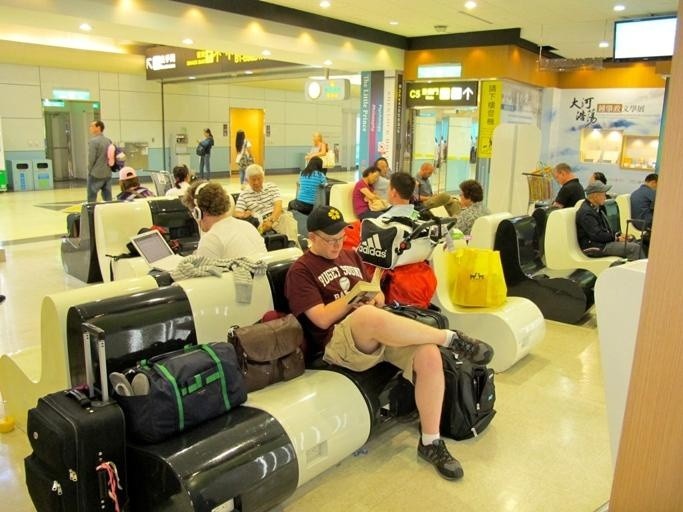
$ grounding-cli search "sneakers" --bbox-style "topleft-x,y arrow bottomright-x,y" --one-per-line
417,436 -> 463,482
448,328 -> 494,365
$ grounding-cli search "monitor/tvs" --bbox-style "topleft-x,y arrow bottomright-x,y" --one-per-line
612,16 -> 677,61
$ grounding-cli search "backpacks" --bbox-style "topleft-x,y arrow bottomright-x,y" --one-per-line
438,345 -> 497,442
105,140 -> 127,173
196,139 -> 208,157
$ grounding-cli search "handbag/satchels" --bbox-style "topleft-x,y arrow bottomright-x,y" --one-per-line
227,311 -> 305,394
342,212 -> 459,312
367,193 -> 393,212
124,224 -> 182,257
262,228 -> 288,251
67,212 -> 80,238
441,238 -> 508,308
107,339 -> 249,444
318,149 -> 336,169
239,140 -> 254,170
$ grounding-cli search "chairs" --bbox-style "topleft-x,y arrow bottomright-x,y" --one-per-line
16,248 -> 451,510
468,167 -> 656,324
291,176 -> 452,285
60,193 -> 319,287
426,238 -> 546,373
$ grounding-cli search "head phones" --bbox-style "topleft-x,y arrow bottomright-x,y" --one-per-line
193,182 -> 212,222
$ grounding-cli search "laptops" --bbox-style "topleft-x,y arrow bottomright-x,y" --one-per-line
131,229 -> 185,272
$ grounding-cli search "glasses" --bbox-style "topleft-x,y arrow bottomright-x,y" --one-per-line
312,230 -> 347,247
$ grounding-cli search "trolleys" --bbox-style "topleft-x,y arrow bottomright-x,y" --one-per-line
520,169 -> 555,216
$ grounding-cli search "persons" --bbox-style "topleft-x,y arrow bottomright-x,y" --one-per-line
549,162 -> 585,211
304,133 -> 329,175
235,130 -> 254,189
87,120 -> 113,204
193,127 -> 213,178
587,172 -> 610,199
282,204 -> 494,480
629,173 -> 659,230
575,181 -> 648,260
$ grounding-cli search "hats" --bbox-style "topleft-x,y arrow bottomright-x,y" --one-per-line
585,179 -> 613,194
306,205 -> 351,237
119,166 -> 137,181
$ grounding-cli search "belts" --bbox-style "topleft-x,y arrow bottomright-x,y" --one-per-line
261,212 -> 273,219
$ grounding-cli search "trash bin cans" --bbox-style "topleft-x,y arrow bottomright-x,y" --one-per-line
6,159 -> 54,192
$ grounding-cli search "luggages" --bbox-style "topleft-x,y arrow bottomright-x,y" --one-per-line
22,384 -> 130,512
381,302 -> 449,330
